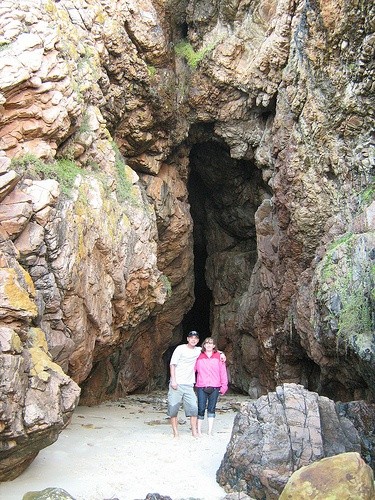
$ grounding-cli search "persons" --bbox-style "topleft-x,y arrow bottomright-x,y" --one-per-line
167,330 -> 226,440
194,337 -> 228,438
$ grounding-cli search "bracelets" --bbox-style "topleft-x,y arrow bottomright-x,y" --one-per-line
220,353 -> 223,354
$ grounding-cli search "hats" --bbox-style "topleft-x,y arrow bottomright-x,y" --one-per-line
188,331 -> 199,338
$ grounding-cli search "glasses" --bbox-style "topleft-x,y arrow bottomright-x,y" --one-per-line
205,342 -> 212,344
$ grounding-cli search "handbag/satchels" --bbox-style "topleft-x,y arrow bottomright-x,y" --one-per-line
203,387 -> 214,394
